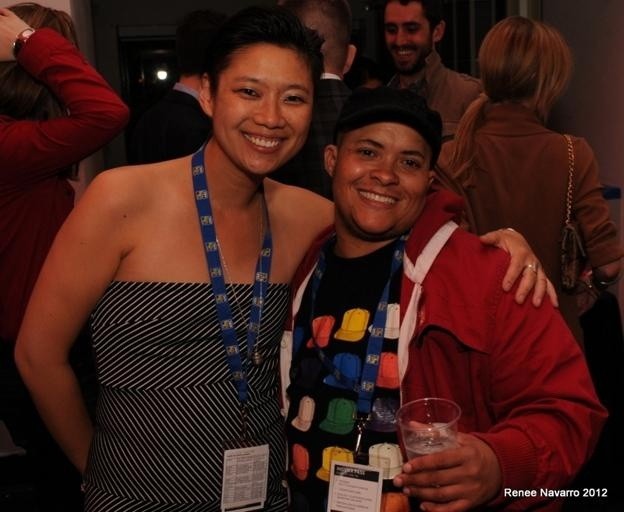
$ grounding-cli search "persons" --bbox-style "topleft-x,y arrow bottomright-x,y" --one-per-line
1,0 -> 624,511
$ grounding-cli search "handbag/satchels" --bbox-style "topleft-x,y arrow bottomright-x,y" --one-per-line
560,133 -> 588,289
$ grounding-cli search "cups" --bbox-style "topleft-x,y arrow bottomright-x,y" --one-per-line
396,398 -> 462,462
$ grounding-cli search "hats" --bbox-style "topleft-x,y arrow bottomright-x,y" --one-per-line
337,85 -> 442,167
